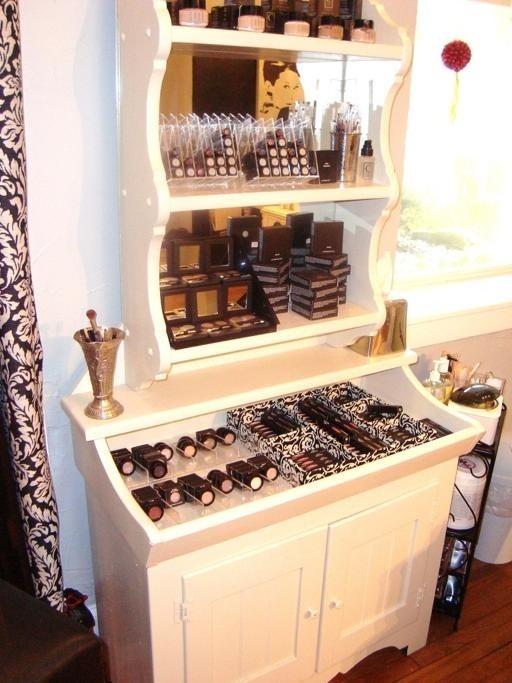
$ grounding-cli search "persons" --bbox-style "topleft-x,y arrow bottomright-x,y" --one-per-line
257,59 -> 304,118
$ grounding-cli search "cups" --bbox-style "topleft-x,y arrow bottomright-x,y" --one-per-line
329,131 -> 361,181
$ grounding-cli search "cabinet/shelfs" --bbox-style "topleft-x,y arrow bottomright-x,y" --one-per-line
60,0 -> 484,683
434,403 -> 507,633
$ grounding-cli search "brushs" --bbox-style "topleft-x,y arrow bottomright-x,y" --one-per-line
79,305 -> 125,342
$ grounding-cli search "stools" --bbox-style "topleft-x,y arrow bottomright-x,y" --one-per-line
0,579 -> 103,683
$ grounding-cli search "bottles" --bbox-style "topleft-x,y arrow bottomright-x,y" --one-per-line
446,455 -> 489,532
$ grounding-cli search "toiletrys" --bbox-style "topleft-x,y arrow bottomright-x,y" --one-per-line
353,138 -> 376,184
167,0 -> 379,45
